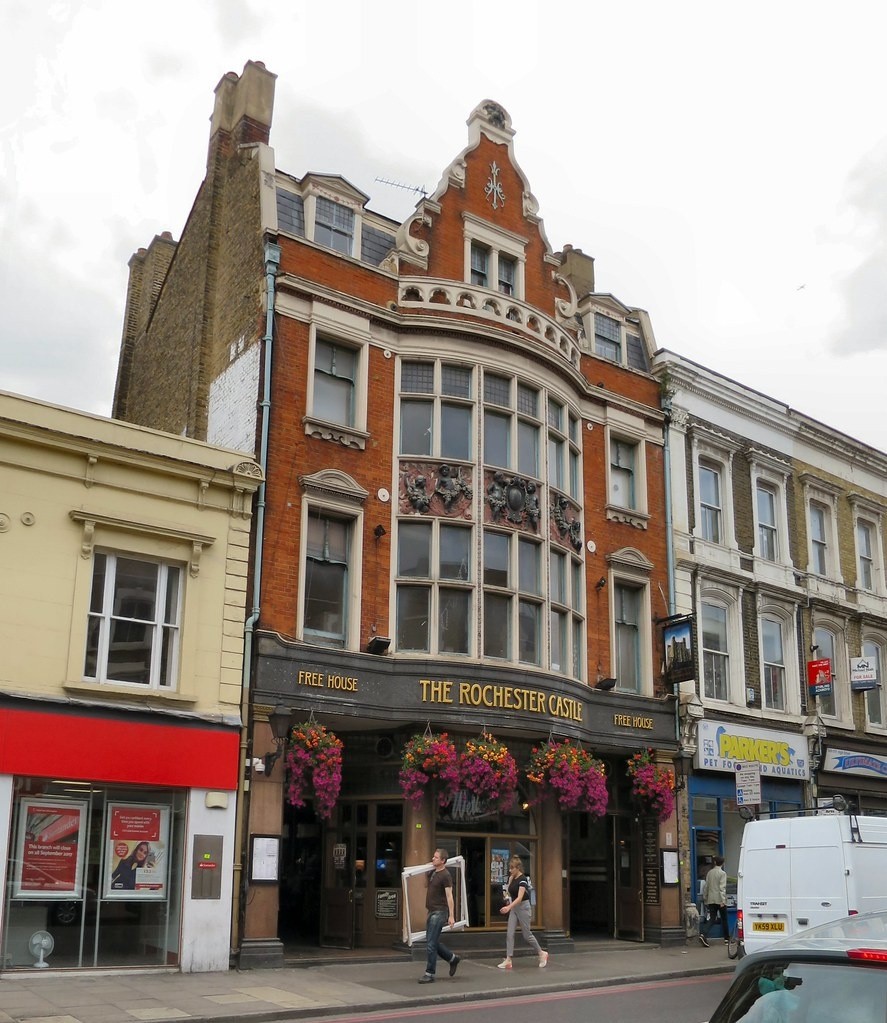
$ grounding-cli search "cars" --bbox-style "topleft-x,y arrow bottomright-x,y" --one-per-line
699,910 -> 887,1023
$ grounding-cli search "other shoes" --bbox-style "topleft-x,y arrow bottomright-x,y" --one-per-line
699,936 -> 710,947
725,941 -> 735,946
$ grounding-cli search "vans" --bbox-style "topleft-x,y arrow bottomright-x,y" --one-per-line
733,793 -> 887,960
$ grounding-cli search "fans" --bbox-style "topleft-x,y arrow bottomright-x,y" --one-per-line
30,931 -> 54,967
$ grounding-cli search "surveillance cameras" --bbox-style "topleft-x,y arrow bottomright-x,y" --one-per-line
255,764 -> 265,774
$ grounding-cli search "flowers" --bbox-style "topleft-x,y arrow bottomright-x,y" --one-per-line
285,718 -> 344,818
398,732 -> 460,808
457,734 -> 520,811
628,748 -> 676,820
522,742 -> 608,818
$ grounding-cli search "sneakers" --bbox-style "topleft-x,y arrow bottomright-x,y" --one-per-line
449,955 -> 462,976
539,951 -> 548,967
418,975 -> 436,984
497,960 -> 512,968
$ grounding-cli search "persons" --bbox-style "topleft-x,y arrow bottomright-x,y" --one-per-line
491,855 -> 504,883
111,842 -> 156,891
417,849 -> 461,984
498,853 -> 548,969
699,856 -> 736,947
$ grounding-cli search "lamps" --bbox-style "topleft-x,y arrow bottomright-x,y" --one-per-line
672,745 -> 692,793
368,637 -> 392,656
265,702 -> 294,778
595,678 -> 618,690
